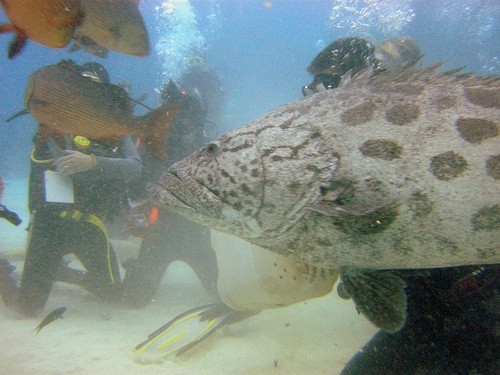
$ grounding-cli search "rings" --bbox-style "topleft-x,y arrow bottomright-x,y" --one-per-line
64,166 -> 70,171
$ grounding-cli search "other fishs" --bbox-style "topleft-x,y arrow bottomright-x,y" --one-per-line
3,59 -> 183,163
29,306 -> 68,338
1,0 -> 150,59
149,50 -> 500,335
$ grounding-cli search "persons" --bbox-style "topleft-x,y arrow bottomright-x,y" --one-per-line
297,37 -> 500,375
104,79 -> 243,316
1,59 -> 130,321
162,40 -> 227,147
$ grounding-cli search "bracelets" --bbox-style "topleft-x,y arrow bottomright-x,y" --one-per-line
90,152 -> 97,167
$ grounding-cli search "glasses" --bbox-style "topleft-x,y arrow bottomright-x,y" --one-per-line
299,69 -> 349,97
186,57 -> 204,67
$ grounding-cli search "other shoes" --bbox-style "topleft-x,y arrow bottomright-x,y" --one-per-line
120,257 -> 139,271
0,259 -> 14,280
